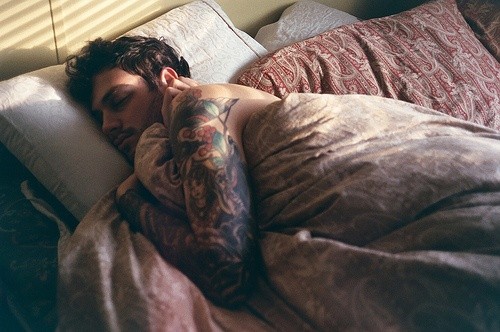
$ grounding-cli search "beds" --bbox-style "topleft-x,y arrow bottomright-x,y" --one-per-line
0,0 -> 500,332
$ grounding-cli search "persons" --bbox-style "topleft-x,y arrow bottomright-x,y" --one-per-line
63,34 -> 285,313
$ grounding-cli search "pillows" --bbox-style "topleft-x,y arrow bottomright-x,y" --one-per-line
0,0 -> 270,223
